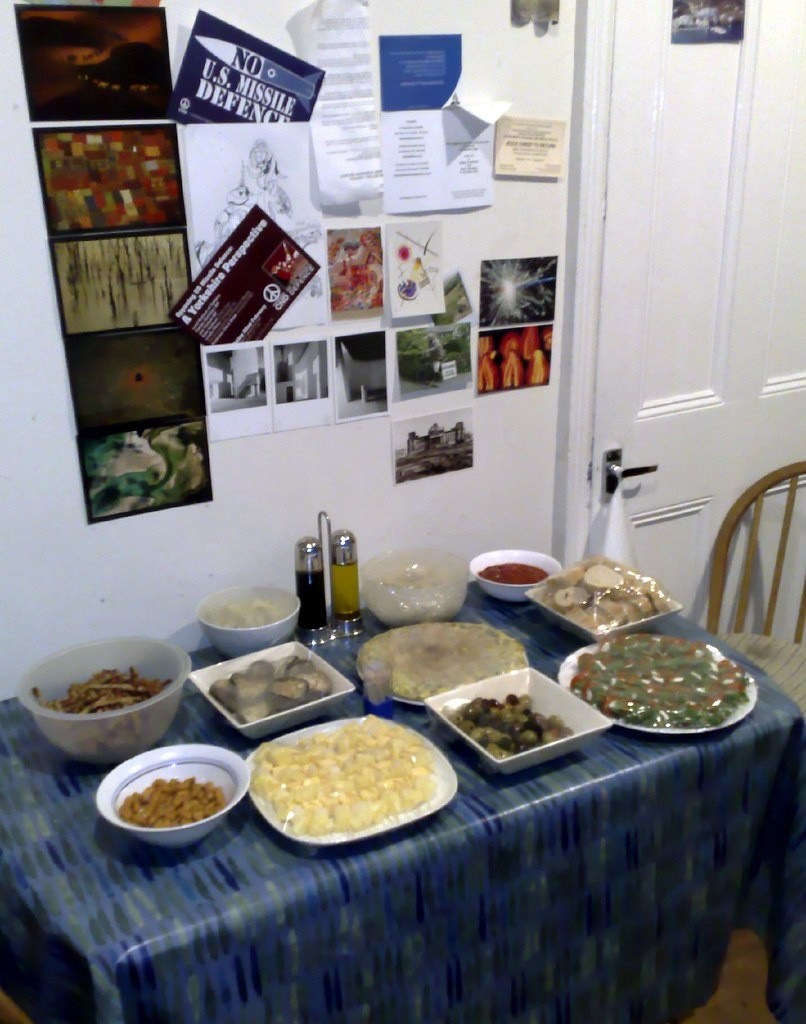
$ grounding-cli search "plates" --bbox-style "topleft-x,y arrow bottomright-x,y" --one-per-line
243,714 -> 460,848
356,623 -> 530,706
556,633 -> 758,733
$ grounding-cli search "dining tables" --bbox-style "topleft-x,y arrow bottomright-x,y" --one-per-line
0,575 -> 806,1024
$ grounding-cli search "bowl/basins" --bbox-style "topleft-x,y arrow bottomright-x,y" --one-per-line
424,666 -> 612,776
362,548 -> 468,626
195,585 -> 301,658
187,640 -> 356,739
471,549 -> 563,602
14,633 -> 192,764
94,743 -> 252,849
523,556 -> 685,645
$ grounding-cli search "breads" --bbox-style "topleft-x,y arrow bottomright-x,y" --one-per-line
539,557 -> 670,632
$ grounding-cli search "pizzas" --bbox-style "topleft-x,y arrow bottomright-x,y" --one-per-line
358,622 -> 526,700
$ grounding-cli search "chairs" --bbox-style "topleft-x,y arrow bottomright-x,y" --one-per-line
703,460 -> 806,724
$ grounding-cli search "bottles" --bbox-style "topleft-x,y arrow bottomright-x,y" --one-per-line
293,536 -> 331,642
331,528 -> 367,634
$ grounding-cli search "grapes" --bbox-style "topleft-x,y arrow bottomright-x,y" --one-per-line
451,693 -> 573,760
